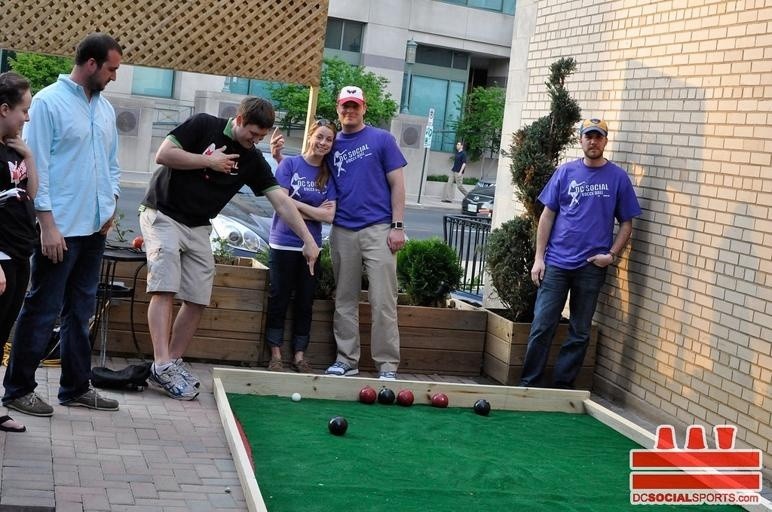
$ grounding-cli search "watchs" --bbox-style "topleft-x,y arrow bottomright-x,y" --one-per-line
390,221 -> 406,231
607,251 -> 617,261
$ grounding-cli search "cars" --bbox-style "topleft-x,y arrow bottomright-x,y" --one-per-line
203,138 -> 411,257
475,177 -> 496,188
460,185 -> 496,221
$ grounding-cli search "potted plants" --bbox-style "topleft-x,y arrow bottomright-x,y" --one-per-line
479,51 -> 601,389
258,233 -> 488,376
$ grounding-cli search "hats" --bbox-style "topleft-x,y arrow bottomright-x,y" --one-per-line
580,118 -> 608,137
337,86 -> 364,107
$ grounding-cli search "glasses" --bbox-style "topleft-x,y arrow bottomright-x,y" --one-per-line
319,120 -> 334,126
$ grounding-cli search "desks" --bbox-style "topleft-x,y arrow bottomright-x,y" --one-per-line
89,246 -> 159,394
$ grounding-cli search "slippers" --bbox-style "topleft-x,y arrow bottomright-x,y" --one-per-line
0,415 -> 26,433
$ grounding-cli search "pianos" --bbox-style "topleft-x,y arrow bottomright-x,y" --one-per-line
329,416 -> 348,435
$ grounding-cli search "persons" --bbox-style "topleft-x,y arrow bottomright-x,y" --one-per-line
1,71 -> 39,432
441,141 -> 469,203
139,96 -> 319,400
1,32 -> 123,416
518,118 -> 643,389
270,86 -> 408,381
264,118 -> 335,374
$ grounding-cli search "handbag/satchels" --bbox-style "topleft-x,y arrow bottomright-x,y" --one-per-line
90,362 -> 153,392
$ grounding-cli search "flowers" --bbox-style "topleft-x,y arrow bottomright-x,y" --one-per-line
110,206 -> 146,252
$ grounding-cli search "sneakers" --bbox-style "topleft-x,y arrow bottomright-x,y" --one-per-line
63,389 -> 119,410
148,362 -> 200,401
291,357 -> 315,373
269,358 -> 283,369
378,371 -> 396,379
324,361 -> 359,376
5,390 -> 56,417
173,357 -> 201,388
